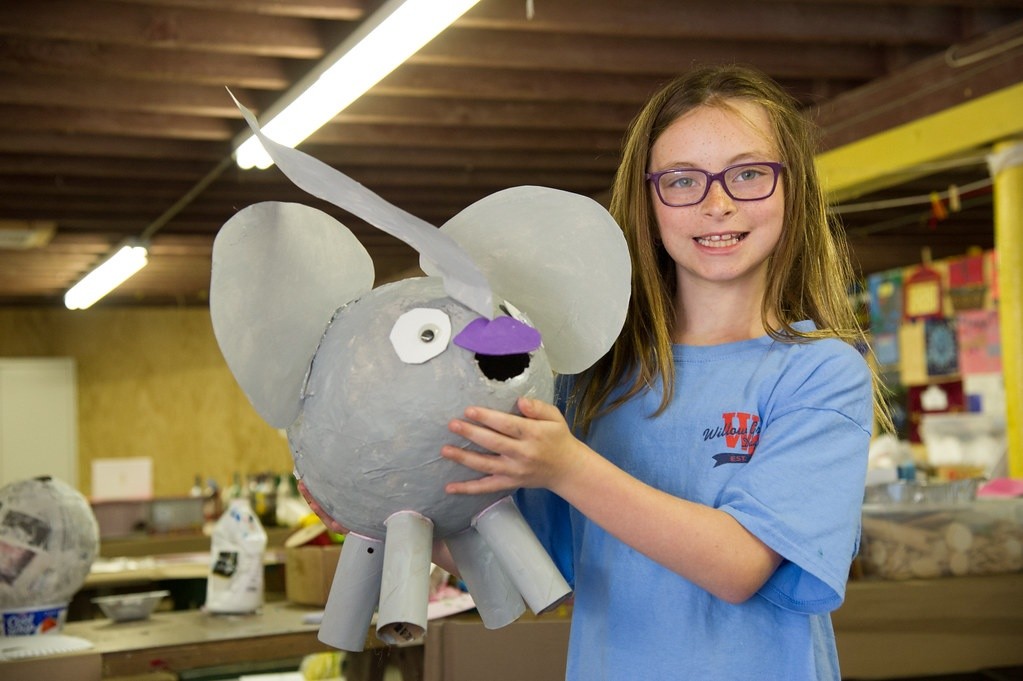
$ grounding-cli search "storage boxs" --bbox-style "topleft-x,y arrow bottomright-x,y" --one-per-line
284,542 -> 343,605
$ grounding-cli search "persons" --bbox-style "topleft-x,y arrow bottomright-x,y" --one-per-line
295,65 -> 899,680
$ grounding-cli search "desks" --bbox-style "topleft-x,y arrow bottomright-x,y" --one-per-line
0,597 -> 392,681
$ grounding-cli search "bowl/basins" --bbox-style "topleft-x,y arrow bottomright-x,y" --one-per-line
1,601 -> 70,636
88,589 -> 168,620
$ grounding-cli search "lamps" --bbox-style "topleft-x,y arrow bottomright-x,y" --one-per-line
64,245 -> 150,315
226,0 -> 481,175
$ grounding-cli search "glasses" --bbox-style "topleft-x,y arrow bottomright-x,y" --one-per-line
646,158 -> 785,207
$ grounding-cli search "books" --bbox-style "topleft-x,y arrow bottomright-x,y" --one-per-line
978,477 -> 1023,501
